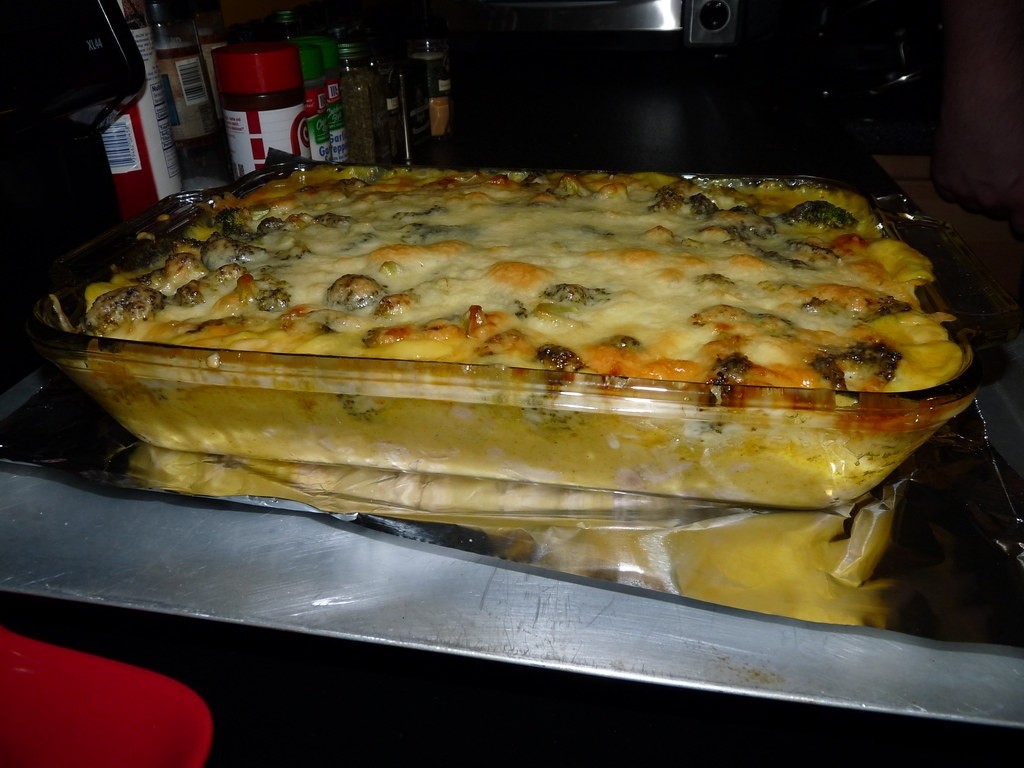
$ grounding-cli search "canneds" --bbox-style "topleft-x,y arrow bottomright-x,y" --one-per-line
211,35 -> 449,181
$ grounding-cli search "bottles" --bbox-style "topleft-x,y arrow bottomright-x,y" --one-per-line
150,0 -> 228,162
278,9 -> 452,167
